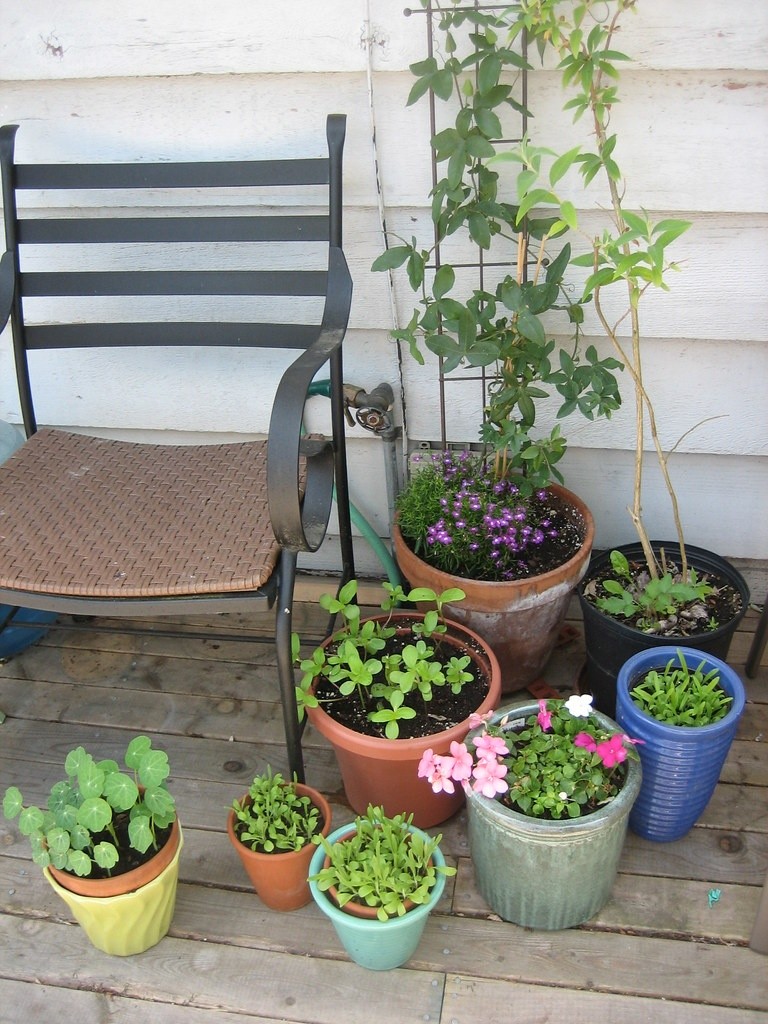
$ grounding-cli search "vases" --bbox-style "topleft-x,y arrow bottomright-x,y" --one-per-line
43,821 -> 184,957
461,699 -> 643,932
392,472 -> 595,695
308,820 -> 447,971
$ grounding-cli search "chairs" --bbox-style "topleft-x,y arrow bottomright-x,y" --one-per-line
0,113 -> 358,784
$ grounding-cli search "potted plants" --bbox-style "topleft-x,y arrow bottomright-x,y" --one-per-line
615,645 -> 745,844
222,765 -> 332,913
306,803 -> 457,923
291,578 -> 502,830
2,736 -> 180,898
574,540 -> 751,723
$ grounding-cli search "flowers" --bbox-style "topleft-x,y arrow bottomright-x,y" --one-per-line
371,0 -> 692,580
416,694 -> 646,820
